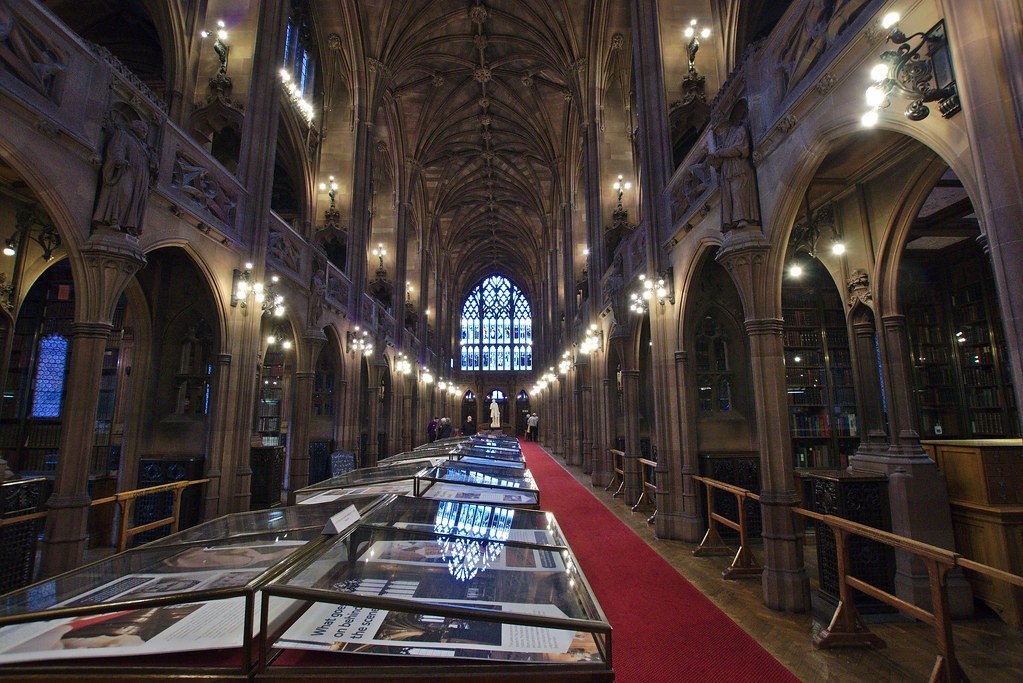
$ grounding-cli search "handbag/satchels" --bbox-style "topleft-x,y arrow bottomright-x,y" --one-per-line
527,424 -> 530,433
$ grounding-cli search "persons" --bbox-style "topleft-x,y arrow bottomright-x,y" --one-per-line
93,120 -> 151,238
702,117 -> 760,232
491,400 -> 499,426
460,416 -> 478,436
523,413 -> 538,443
427,418 -> 453,442
309,270 -> 327,326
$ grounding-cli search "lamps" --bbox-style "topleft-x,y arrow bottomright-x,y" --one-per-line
4,206 -> 62,262
786,187 -> 845,277
684,19 -> 710,71
319,175 -> 340,220
528,266 -> 675,397
613,175 -> 630,220
860,12 -> 963,129
201,21 -> 232,91
231,279 -> 460,397
372,243 -> 386,281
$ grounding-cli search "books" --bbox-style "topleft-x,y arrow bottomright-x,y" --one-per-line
258,352 -> 284,446
901,269 -> 1021,438
314,376 -> 333,415
176,343 -> 205,417
780,289 -> 859,471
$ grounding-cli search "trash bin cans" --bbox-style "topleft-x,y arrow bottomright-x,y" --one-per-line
809,468 -> 899,612
250,446 -> 282,511
616,436 -> 651,489
308,439 -> 335,485
0,473 -> 49,618
130,454 -> 205,548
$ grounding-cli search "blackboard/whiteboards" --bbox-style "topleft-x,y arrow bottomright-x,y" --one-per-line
330,450 -> 355,478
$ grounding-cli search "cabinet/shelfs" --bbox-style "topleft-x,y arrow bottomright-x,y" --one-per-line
778,278 -> 1022,546
0,300 -> 127,542
920,440 -> 1023,630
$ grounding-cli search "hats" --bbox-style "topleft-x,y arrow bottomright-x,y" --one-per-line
526,414 -> 530,416
532,413 -> 536,416
